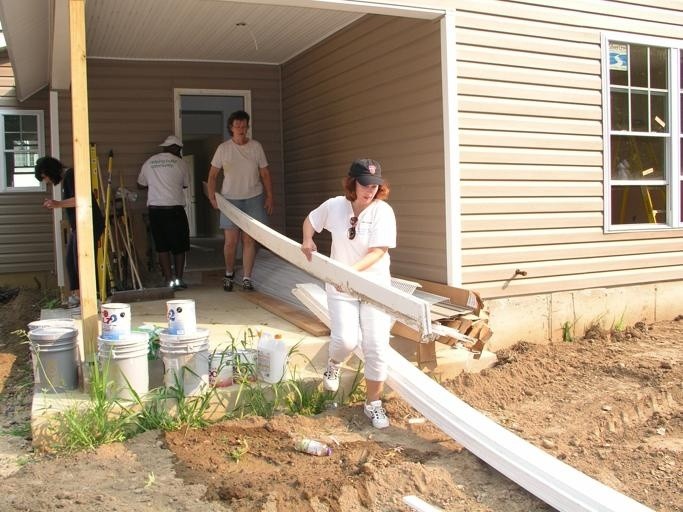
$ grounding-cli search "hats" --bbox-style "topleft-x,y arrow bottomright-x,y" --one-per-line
349,159 -> 384,186
160,135 -> 183,147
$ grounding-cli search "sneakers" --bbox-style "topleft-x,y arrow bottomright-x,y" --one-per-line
324,358 -> 340,391
166,281 -> 175,290
61,295 -> 81,307
175,278 -> 187,288
224,272 -> 234,292
364,399 -> 389,428
242,279 -> 253,291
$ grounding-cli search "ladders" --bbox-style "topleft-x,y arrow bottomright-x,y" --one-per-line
613,128 -> 666,223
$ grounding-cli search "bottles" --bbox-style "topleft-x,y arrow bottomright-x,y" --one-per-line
295,439 -> 331,457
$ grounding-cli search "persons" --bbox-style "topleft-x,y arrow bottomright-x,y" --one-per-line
34,156 -> 105,308
137,135 -> 189,289
300,158 -> 397,430
208,110 -> 273,292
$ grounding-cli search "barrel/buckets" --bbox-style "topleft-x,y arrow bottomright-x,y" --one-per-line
256,333 -> 284,386
98,334 -> 150,403
26,319 -> 78,330
156,327 -> 211,397
26,329 -> 79,396
